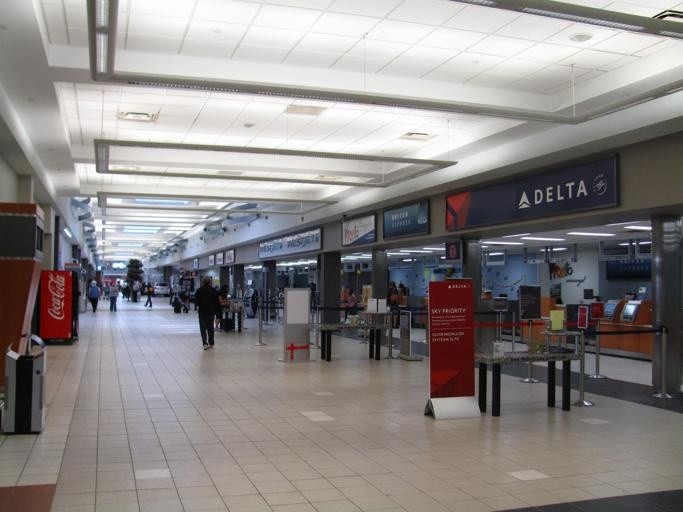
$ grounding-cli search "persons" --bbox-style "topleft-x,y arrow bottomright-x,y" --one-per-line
339,285 -> 371,324
388,280 -> 399,328
193,276 -> 222,350
88,276 -> 317,331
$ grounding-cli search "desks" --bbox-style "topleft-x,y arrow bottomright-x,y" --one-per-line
471,345 -> 582,416
308,318 -> 390,362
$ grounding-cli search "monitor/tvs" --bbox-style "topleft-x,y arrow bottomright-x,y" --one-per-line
624,304 -> 635,315
604,303 -> 614,313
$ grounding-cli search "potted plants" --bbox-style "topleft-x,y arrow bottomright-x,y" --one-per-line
525,336 -> 545,354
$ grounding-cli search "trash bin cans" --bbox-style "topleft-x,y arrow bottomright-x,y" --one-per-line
78,296 -> 88,313
1,334 -> 47,434
